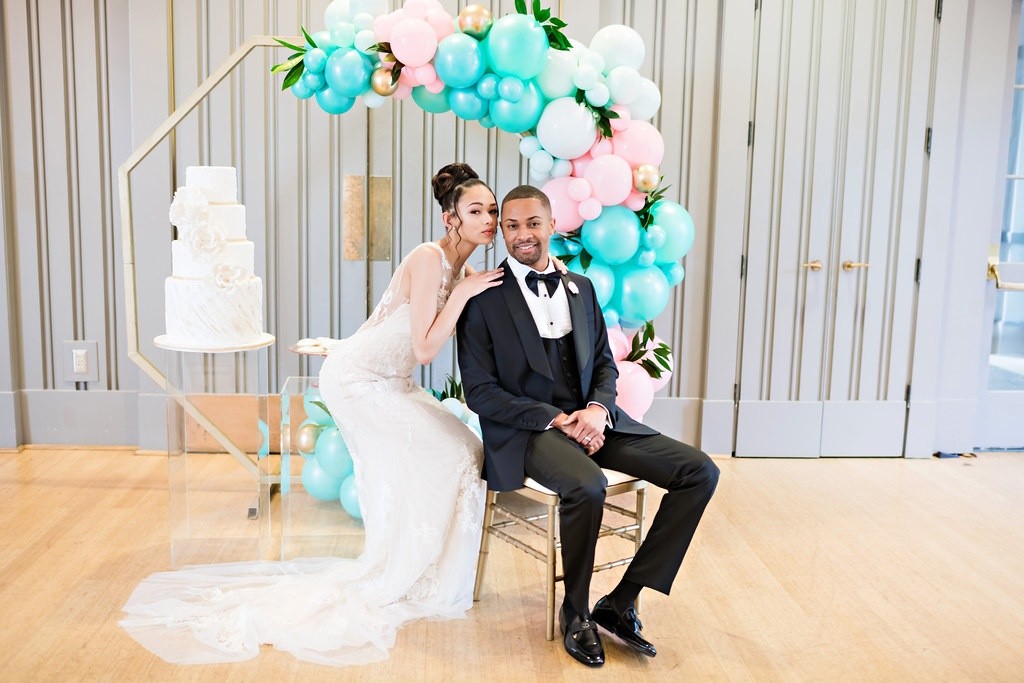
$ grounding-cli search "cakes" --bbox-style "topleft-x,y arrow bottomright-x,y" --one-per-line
164,165 -> 262,345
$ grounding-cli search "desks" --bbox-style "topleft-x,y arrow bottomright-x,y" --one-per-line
278,372 -> 406,562
151,328 -> 277,569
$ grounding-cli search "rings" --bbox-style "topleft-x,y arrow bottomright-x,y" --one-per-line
585,437 -> 591,442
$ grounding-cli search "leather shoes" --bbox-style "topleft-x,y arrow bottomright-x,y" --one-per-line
590,595 -> 657,658
559,604 -> 605,666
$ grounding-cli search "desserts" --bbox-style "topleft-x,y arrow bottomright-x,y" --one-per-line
297,336 -> 336,352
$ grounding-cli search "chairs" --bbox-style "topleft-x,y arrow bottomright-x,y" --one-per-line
466,437 -> 648,641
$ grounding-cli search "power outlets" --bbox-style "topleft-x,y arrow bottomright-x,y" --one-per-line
72,348 -> 89,375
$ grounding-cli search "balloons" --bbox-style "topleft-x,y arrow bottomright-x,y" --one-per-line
442,397 -> 482,438
296,393 -> 362,519
292,0 -> 694,423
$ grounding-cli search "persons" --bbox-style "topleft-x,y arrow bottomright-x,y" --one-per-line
457,185 -> 719,666
121,164 -> 570,666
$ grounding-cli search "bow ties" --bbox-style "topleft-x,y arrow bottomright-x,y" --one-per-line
525,270 -> 562,298
